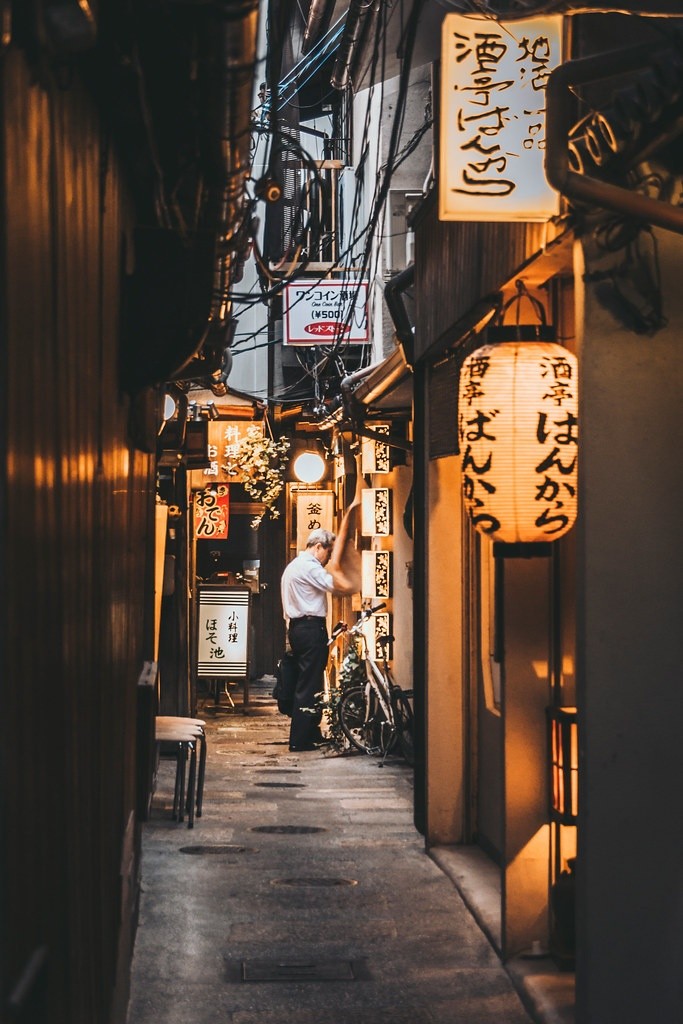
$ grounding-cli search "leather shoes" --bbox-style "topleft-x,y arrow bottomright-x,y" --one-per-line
288,743 -> 317,752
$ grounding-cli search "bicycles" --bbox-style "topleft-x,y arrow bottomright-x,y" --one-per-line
326,601 -> 415,769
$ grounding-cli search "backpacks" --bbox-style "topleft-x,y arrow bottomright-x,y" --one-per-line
274,658 -> 293,718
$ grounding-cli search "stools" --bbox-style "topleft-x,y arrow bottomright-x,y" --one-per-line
144,715 -> 207,829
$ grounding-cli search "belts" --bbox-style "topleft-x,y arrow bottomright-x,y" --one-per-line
294,616 -> 326,622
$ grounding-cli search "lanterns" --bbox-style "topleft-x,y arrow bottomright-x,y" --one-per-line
457,293 -> 579,558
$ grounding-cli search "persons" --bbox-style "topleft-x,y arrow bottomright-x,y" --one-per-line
280,529 -> 356,752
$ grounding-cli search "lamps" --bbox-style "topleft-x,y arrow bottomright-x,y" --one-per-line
290,438 -> 328,484
